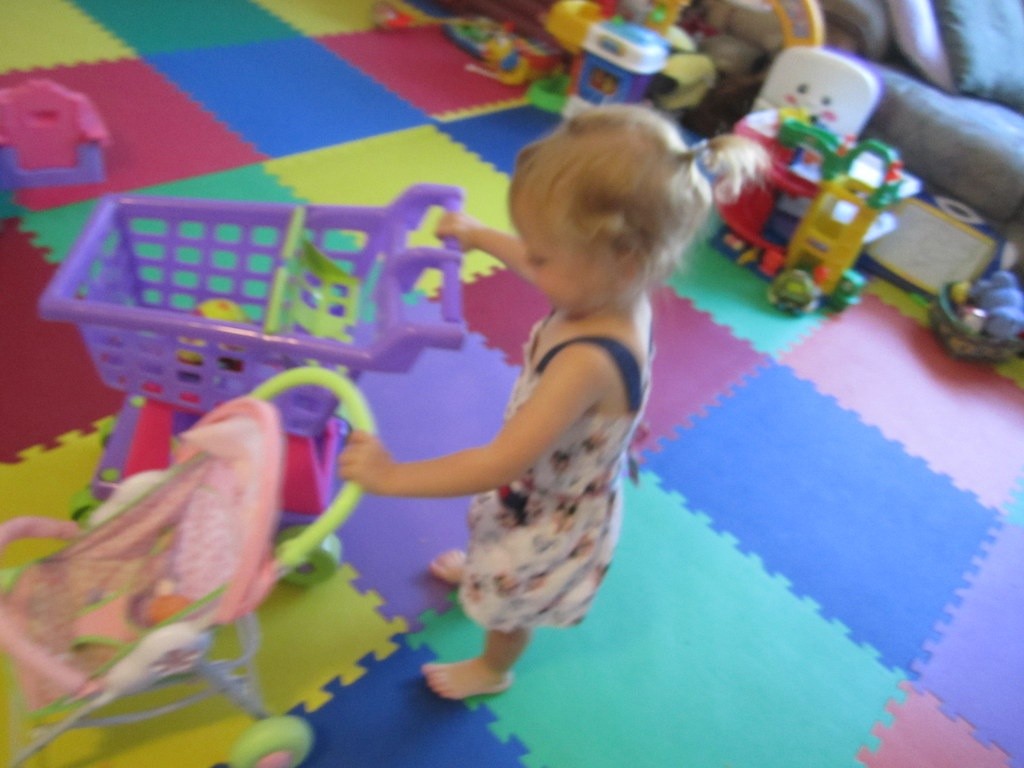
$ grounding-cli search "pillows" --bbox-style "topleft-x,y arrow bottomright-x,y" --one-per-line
936,0 -> 1024,109
821,0 -> 1024,223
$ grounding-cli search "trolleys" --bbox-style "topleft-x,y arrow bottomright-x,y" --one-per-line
39,182 -> 474,530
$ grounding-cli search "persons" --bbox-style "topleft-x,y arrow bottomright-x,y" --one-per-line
336,107 -> 769,700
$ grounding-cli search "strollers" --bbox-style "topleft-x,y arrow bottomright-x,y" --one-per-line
0,367 -> 380,768
